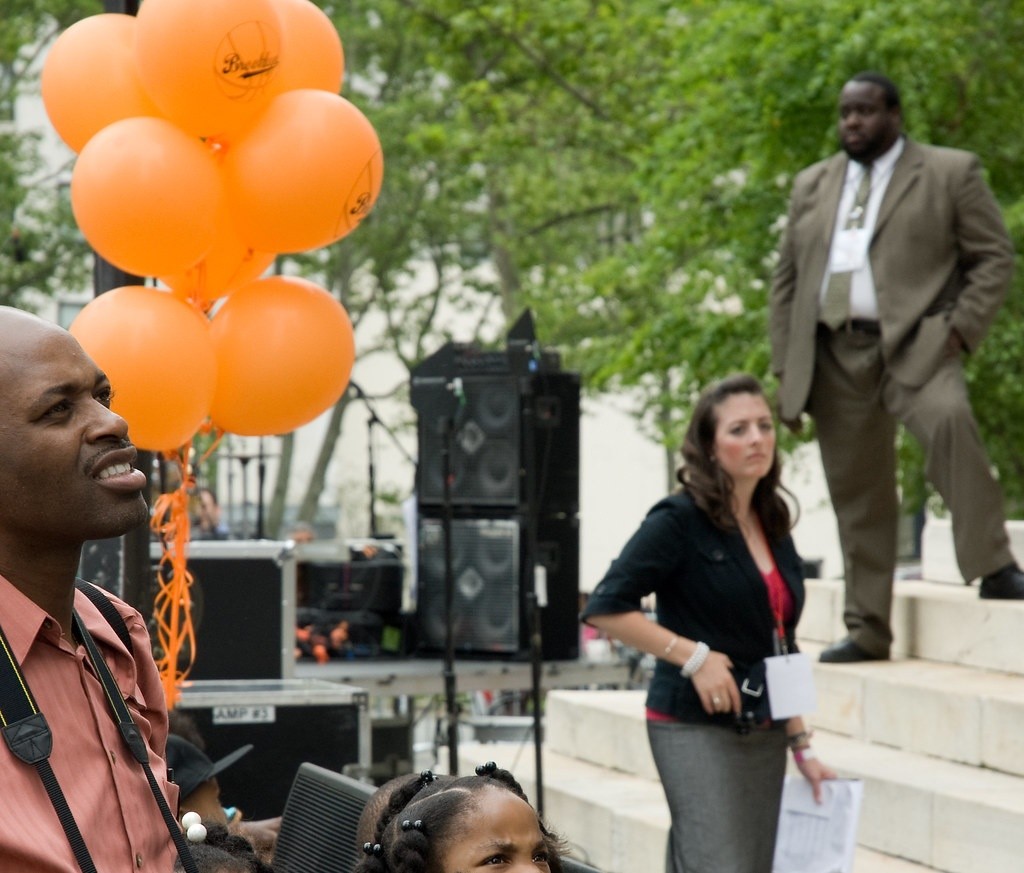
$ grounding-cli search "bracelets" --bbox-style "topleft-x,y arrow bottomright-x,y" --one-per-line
680,641 -> 710,679
658,636 -> 679,663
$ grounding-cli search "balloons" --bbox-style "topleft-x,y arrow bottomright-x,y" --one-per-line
42,0 -> 384,454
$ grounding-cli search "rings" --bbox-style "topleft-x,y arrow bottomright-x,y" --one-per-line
712,697 -> 720,704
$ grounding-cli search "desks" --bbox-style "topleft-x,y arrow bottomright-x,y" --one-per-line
297,659 -> 631,694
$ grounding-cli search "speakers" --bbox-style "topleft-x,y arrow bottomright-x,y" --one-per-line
407,372 -> 580,666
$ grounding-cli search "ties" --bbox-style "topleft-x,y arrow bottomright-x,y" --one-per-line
820,165 -> 872,329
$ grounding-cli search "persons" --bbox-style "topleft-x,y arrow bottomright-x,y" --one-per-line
770,70 -> 1024,661
0,304 -> 188,873
163,712 -> 285,873
351,760 -> 569,873
183,488 -> 233,540
578,374 -> 839,873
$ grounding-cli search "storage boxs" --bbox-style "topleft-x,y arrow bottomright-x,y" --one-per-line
150,538 -> 302,679
163,683 -> 373,820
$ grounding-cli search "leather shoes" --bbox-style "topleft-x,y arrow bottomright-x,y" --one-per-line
979,567 -> 1024,600
819,642 -> 889,662
